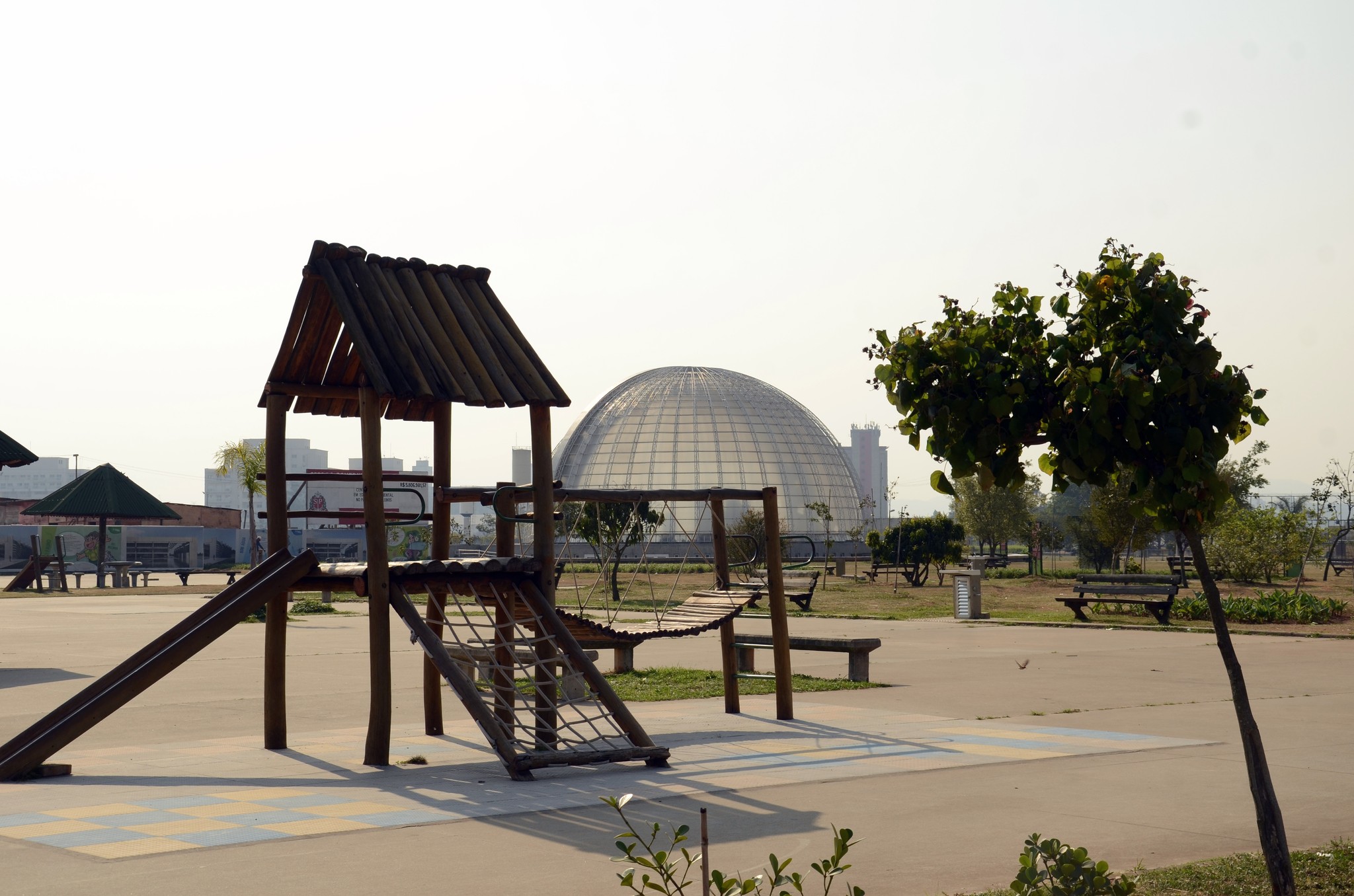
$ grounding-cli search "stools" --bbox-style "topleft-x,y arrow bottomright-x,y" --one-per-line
96,571 -> 152,588
45,573 -> 84,588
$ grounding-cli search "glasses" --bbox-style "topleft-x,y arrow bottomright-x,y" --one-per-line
259,539 -> 260,541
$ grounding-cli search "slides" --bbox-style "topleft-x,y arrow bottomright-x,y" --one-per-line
0,548 -> 320,783
3,561 -> 51,592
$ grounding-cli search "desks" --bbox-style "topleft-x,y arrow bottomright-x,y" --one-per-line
123,562 -> 142,586
48,562 -> 73,587
101,561 -> 137,588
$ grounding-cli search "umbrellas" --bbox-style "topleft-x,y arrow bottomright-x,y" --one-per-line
0,432 -> 40,472
20,463 -> 183,585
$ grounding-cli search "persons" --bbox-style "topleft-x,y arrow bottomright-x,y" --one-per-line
250,537 -> 266,561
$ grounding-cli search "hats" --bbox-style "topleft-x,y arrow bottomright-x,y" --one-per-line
257,536 -> 261,540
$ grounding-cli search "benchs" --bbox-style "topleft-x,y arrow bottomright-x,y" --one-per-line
440,644 -> 599,701
735,632 -> 881,683
958,553 -> 1011,571
803,566 -> 836,575
1055,574 -> 1182,626
726,569 -> 820,612
862,563 -> 915,583
175,571 -> 241,586
1166,556 -> 1231,581
1330,558 -> 1354,576
